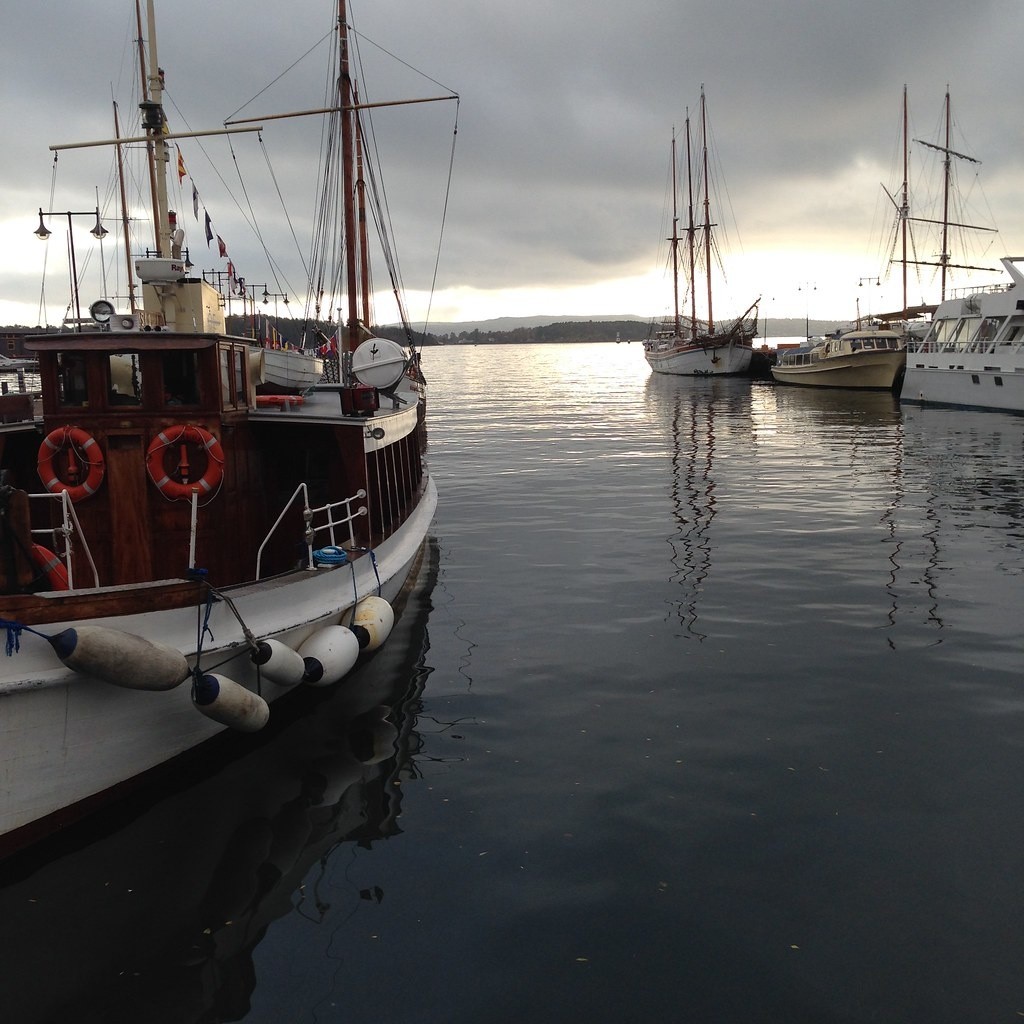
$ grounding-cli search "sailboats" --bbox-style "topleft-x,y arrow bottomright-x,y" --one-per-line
643,83 -> 766,380
0,0 -> 465,842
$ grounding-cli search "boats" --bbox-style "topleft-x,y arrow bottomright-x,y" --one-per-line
770,297 -> 907,390
824,80 -> 1024,414
246,348 -> 324,394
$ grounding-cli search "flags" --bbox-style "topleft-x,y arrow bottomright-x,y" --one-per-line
205,209 -> 214,250
162,117 -> 170,136
178,146 -> 187,186
271,326 -> 288,351
259,314 -> 270,349
216,233 -> 228,258
226,257 -> 237,295
238,277 -> 246,296
192,180 -> 198,221
292,331 -> 338,360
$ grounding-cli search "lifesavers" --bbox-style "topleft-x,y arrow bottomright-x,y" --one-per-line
146,425 -> 225,506
29,544 -> 67,590
257,395 -> 304,407
36,425 -> 104,504
